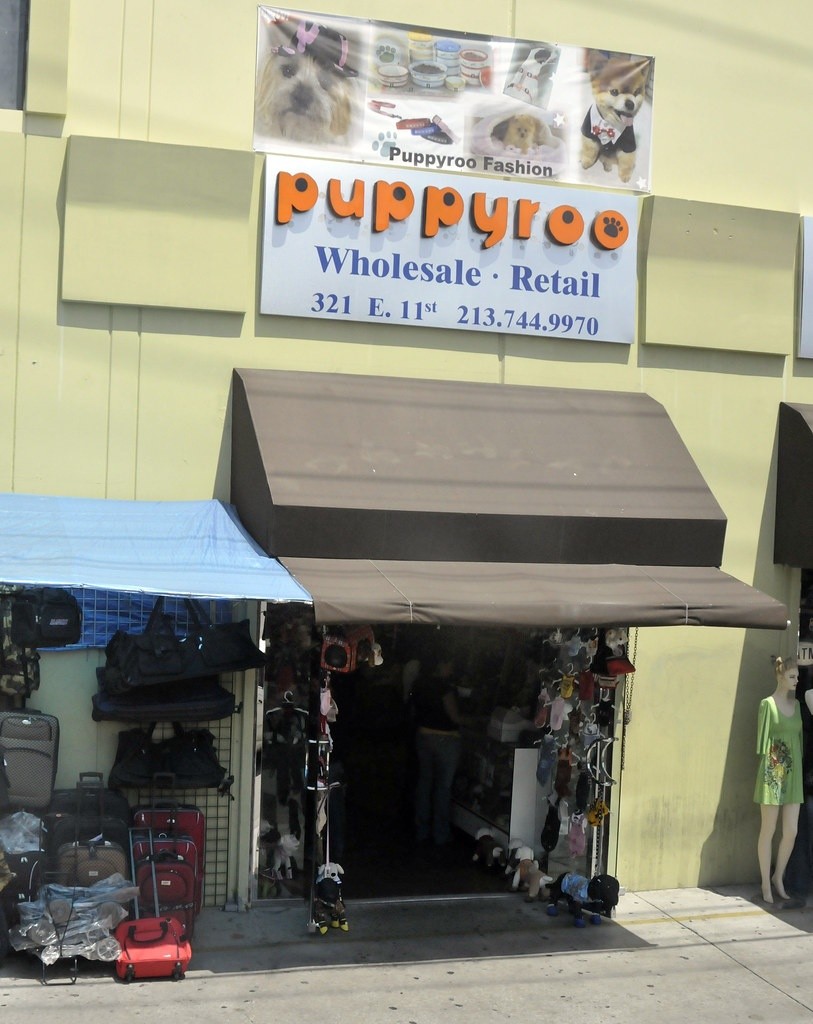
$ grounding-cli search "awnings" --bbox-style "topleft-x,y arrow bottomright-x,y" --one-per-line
0,369 -> 813,629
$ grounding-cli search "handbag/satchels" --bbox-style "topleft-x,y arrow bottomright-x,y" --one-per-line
104,596 -> 268,696
10,588 -> 82,649
108,721 -> 226,790
92,665 -> 237,723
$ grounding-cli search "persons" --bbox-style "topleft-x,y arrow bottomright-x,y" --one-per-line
756,654 -> 806,902
408,648 -> 478,850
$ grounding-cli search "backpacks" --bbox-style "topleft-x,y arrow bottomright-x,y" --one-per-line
0,585 -> 41,695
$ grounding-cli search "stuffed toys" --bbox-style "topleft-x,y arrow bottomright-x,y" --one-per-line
274,834 -> 619,936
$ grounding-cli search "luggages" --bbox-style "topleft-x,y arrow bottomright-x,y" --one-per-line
0,707 -> 205,983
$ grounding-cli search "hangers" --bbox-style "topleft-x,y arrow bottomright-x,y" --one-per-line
266,690 -> 309,715
533,627 -> 621,813
